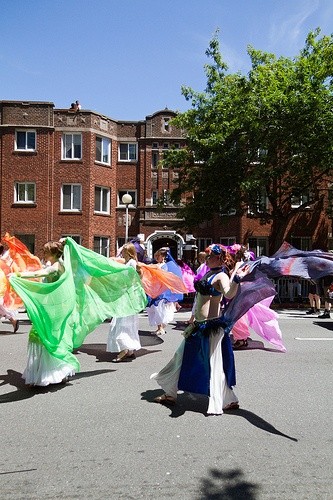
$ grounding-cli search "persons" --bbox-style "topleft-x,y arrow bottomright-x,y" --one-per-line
306,276 -> 333,318
0,241 -> 254,412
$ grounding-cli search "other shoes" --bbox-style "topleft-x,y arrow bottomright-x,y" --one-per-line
112,350 -> 136,363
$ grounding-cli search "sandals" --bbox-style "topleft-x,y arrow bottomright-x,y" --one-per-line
232,339 -> 248,349
154,393 -> 176,403
183,321 -> 194,325
223,402 -> 240,413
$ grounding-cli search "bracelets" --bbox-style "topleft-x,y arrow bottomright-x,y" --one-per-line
14,271 -> 20,277
232,275 -> 240,284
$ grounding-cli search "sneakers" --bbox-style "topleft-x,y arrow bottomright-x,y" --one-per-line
318,309 -> 330,318
14,320 -> 20,332
151,328 -> 167,335
314,309 -> 321,314
306,307 -> 316,315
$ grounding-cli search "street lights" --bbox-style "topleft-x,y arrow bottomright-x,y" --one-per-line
122,192 -> 132,243
